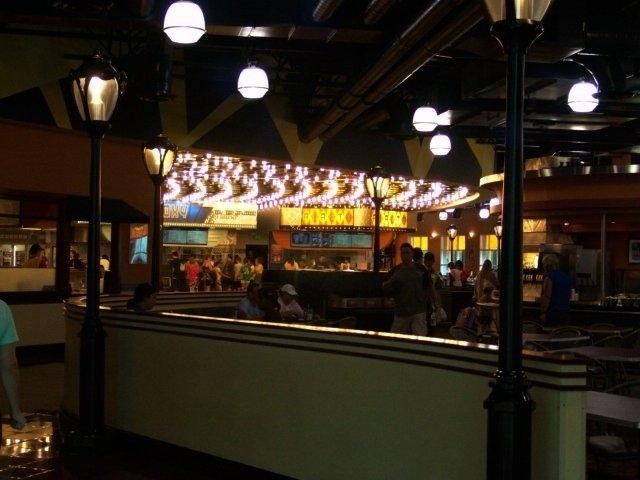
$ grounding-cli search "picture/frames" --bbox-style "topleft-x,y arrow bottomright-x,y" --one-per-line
626,238 -> 640,264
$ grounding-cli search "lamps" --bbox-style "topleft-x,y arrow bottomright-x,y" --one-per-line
438,209 -> 449,222
429,127 -> 452,157
236,56 -> 272,101
478,205 -> 490,219
411,94 -> 439,134
163,0 -> 209,48
565,72 -> 602,115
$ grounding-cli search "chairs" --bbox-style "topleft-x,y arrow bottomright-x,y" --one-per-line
320,315 -> 358,329
234,304 -> 245,320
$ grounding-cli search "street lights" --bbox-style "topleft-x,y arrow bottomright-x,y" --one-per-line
493,220 -> 502,280
61,50 -> 129,472
363,159 -> 393,270
140,133 -> 179,294
484,0 -> 551,478
444,223 -> 459,289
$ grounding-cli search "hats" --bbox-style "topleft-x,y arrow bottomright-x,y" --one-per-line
282,284 -> 298,295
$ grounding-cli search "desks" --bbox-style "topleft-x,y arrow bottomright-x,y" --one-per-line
281,316 -> 335,328
450,301 -> 640,398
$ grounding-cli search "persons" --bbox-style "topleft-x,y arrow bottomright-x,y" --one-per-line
0,299 -> 26,430
